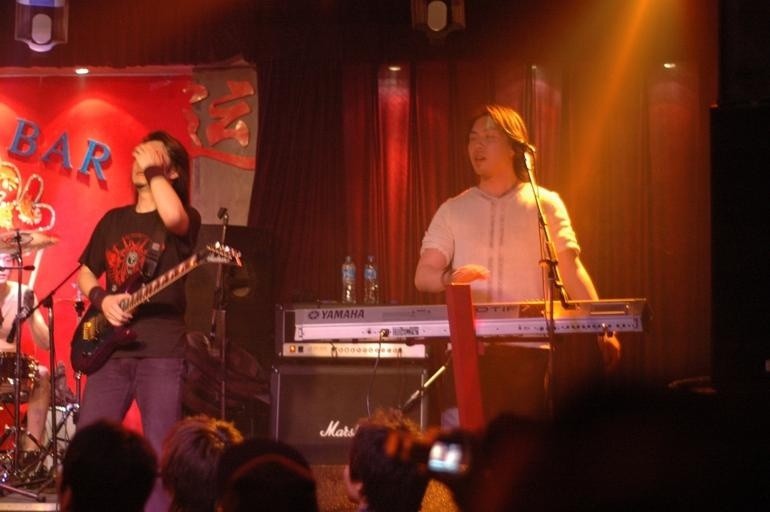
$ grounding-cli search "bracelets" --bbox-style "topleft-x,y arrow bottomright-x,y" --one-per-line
88,285 -> 110,310
142,167 -> 163,181
440,269 -> 447,288
448,270 -> 454,286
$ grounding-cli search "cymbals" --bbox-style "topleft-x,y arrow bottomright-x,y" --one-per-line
0,230 -> 57,253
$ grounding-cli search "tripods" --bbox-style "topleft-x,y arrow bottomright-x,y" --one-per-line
0,323 -> 83,502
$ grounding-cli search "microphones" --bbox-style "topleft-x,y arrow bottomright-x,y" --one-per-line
518,145 -> 532,170
400,358 -> 451,414
2,264 -> 36,271
218,205 -> 231,224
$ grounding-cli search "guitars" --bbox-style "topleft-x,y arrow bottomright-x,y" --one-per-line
71,241 -> 242,376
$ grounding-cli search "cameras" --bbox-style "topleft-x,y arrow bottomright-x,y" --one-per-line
429,441 -> 466,471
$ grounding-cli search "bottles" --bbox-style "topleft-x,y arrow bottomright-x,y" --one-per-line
363,256 -> 380,306
341,256 -> 357,306
56,360 -> 66,388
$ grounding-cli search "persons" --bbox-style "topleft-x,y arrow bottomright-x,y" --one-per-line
54,370 -> 722,510
0,254 -> 55,474
412,103 -> 621,423
75,128 -> 201,471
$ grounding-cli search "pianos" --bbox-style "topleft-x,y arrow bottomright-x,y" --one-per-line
293,298 -> 652,342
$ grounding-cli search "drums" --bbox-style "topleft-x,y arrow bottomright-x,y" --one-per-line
0,351 -> 39,403
12,396 -> 82,494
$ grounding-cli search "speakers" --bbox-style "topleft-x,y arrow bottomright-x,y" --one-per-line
270,361 -> 427,464
